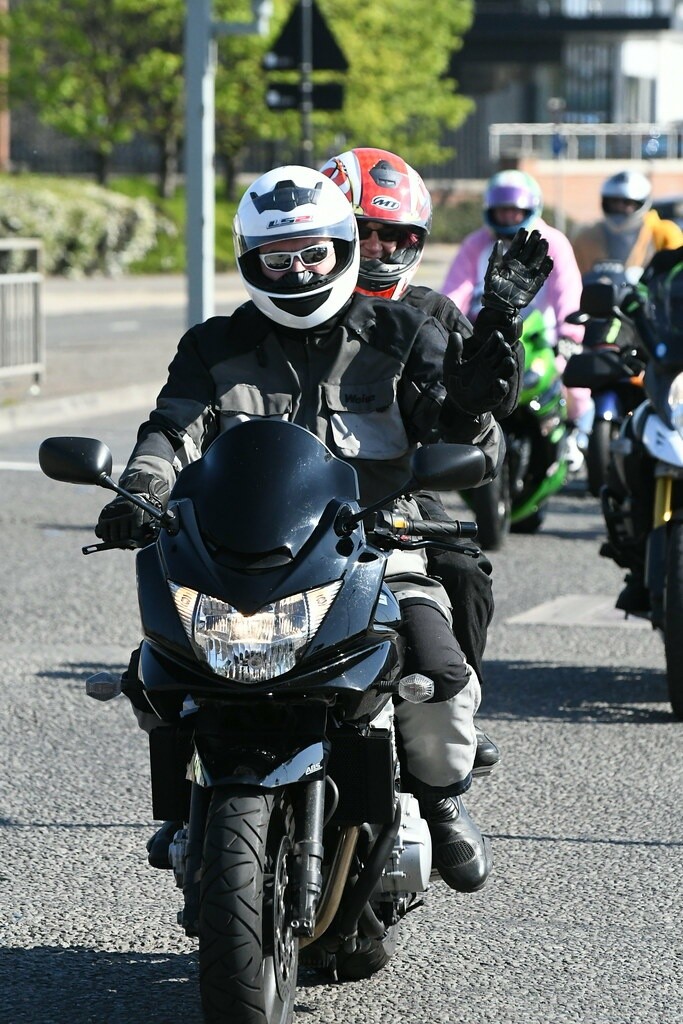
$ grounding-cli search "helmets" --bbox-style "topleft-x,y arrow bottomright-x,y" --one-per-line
231,164 -> 360,330
482,170 -> 543,238
317,147 -> 433,301
601,171 -> 653,238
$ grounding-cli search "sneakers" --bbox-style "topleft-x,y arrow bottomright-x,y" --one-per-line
421,792 -> 493,893
472,723 -> 499,778
148,819 -> 185,869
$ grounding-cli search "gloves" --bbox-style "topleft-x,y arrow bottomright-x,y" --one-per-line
95,472 -> 170,550
441,331 -> 517,416
482,227 -> 554,315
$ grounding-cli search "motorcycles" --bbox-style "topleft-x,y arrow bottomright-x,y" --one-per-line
467,291 -> 572,537
565,262 -> 651,493
603,279 -> 683,722
37,417 -> 504,1024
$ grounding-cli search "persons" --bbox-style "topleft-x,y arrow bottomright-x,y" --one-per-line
588,235 -> 680,623
562,172 -> 682,276
428,167 -> 589,532
292,148 -> 526,776
70,158 -> 559,898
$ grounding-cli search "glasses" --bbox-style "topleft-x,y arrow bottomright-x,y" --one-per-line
256,241 -> 334,272
357,224 -> 406,241
